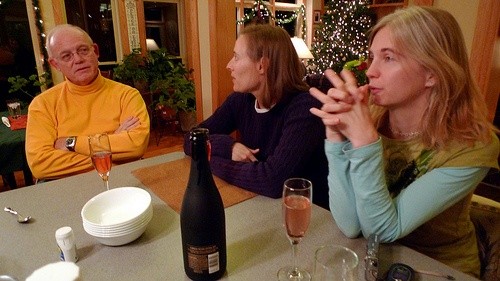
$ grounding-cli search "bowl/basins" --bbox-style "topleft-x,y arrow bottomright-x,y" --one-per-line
81,186 -> 154,247
24,261 -> 80,281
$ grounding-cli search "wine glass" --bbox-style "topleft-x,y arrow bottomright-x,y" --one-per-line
277,178 -> 313,281
88,133 -> 113,191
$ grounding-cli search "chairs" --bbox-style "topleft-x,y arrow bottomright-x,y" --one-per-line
470,181 -> 500,281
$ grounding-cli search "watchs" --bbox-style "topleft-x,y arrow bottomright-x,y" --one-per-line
66,136 -> 76,152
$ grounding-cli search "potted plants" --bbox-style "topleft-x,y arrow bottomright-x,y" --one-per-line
111,44 -> 198,122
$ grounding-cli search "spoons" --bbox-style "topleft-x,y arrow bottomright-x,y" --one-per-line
4,206 -> 31,223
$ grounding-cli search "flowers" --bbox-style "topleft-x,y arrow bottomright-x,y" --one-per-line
344,57 -> 370,86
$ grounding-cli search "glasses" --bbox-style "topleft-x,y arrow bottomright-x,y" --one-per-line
54,46 -> 93,62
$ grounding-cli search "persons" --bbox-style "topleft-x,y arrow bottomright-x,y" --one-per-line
184,23 -> 330,209
25,24 -> 150,185
309,6 -> 500,276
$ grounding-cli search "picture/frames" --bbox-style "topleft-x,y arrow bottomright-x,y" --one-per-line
313,9 -> 322,24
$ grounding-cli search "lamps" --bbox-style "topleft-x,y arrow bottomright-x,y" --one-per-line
290,36 -> 314,60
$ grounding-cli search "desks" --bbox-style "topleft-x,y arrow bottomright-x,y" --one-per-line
0,110 -> 33,189
0,152 -> 478,281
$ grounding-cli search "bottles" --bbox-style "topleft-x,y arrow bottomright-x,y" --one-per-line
180,129 -> 228,280
55,226 -> 78,263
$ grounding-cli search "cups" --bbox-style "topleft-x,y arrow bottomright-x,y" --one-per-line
310,245 -> 359,281
